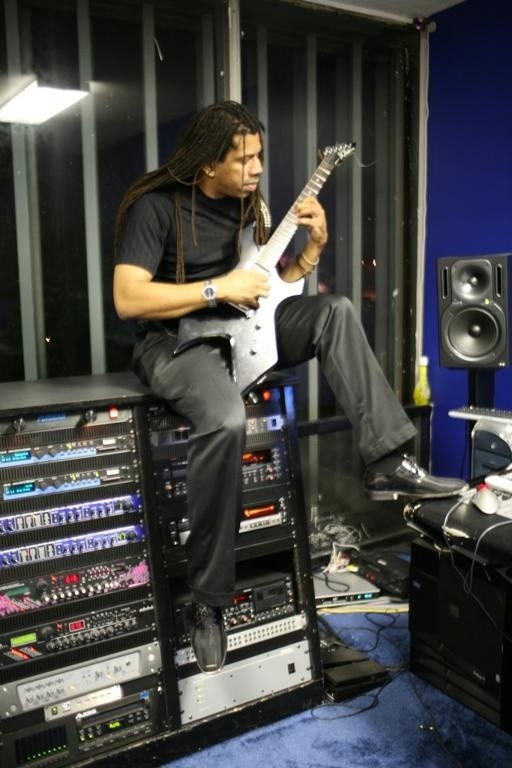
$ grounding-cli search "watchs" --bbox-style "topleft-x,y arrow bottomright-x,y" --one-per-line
202,278 -> 217,309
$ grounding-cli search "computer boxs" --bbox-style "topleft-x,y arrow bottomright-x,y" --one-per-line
468,421 -> 512,477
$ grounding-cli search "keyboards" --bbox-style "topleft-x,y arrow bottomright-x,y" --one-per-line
448,403 -> 512,426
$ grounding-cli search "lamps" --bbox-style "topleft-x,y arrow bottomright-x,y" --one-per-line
0,71 -> 91,126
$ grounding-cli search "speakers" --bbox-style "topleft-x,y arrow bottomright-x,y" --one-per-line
436,253 -> 511,368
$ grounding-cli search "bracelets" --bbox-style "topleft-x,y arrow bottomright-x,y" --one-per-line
295,254 -> 316,275
301,249 -> 319,265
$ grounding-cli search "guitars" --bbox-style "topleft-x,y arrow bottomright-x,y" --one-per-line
172,143 -> 353,396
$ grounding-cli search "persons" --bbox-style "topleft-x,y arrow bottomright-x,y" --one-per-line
109,99 -> 471,677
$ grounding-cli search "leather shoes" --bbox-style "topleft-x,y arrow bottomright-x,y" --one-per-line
190,599 -> 227,674
364,453 -> 468,501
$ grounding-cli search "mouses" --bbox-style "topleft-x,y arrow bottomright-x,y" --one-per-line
471,487 -> 500,514
